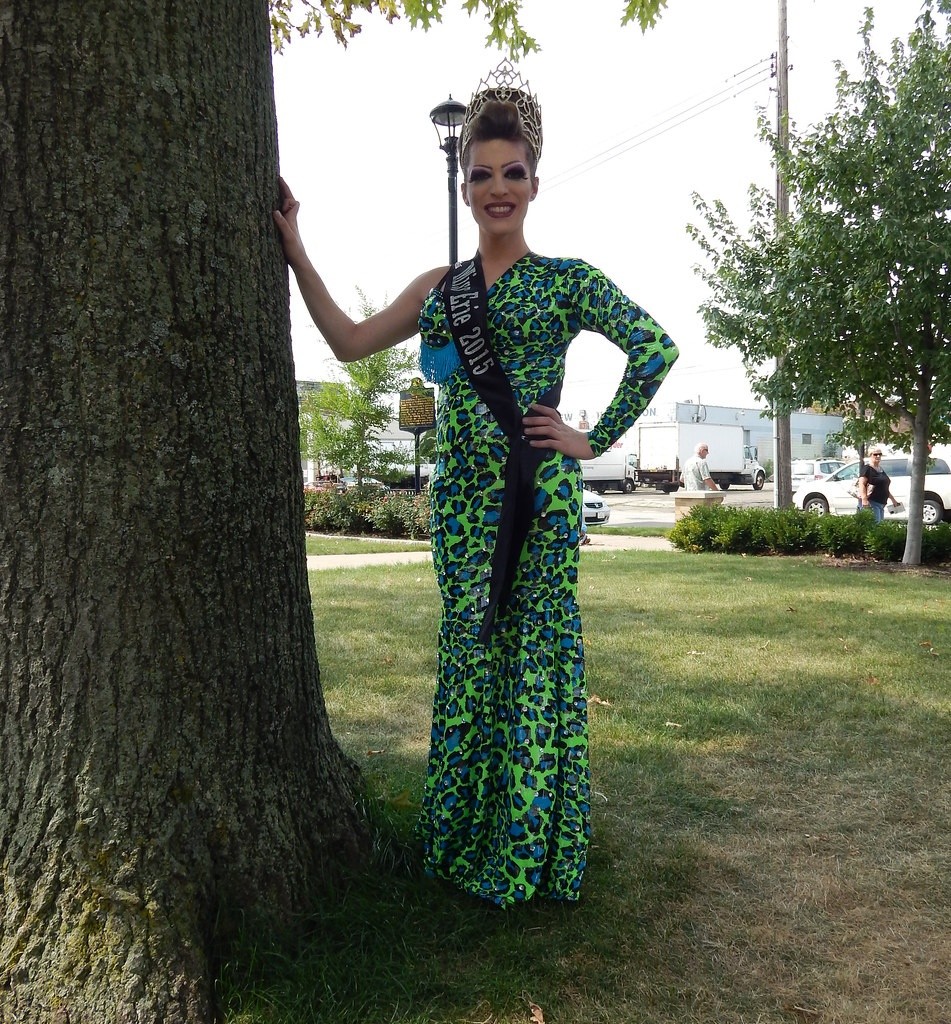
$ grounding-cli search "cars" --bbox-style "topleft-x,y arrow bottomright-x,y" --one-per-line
582,488 -> 611,526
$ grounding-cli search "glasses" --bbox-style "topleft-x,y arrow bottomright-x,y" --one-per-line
873,453 -> 882,456
704,448 -> 708,452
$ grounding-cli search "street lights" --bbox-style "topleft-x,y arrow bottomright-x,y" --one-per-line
428,95 -> 471,267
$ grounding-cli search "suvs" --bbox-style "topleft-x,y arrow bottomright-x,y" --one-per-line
791,458 -> 848,494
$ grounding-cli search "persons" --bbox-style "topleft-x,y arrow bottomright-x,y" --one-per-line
272,57 -> 679,912
679,443 -> 719,491
859,447 -> 898,523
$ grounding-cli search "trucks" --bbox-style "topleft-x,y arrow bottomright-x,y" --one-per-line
635,422 -> 766,493
793,455 -> 951,526
576,433 -> 639,494
